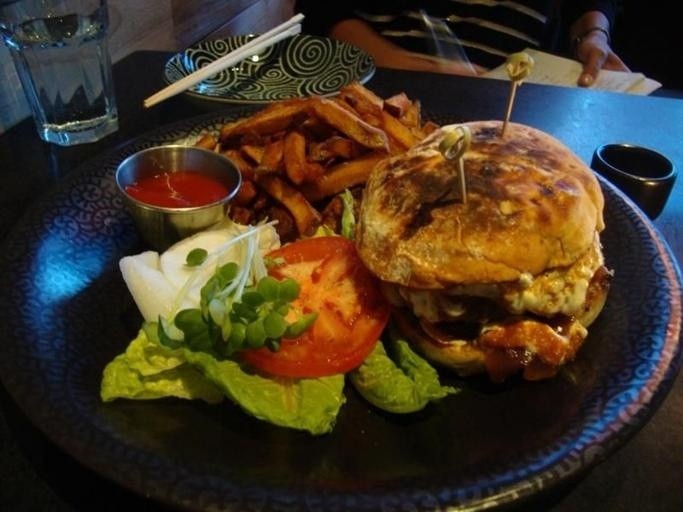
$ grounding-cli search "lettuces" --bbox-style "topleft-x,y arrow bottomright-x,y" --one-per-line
100,190 -> 463,434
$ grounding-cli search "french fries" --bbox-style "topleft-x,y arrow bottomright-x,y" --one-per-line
191,80 -> 439,239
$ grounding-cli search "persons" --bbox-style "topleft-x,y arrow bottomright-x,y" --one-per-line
294,1 -> 631,86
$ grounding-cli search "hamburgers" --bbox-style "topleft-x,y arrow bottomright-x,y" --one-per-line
355,119 -> 610,370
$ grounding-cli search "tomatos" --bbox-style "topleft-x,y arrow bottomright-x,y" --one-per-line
241,236 -> 391,377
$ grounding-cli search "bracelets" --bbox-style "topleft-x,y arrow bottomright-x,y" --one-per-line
569,28 -> 611,56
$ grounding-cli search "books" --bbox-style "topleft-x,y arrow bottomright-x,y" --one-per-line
480,47 -> 663,96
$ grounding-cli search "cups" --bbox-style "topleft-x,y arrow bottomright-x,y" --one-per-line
0,1 -> 119,150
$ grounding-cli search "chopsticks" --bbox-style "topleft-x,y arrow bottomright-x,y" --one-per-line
141,13 -> 306,109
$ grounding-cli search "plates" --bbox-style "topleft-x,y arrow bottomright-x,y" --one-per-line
6,101 -> 683,509
163,32 -> 378,108
